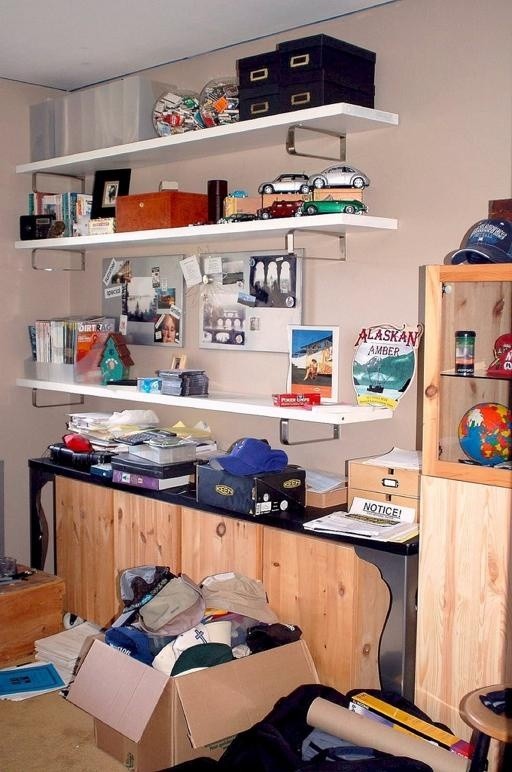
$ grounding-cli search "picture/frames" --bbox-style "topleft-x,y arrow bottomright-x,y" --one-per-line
286,323 -> 340,405
91,168 -> 132,220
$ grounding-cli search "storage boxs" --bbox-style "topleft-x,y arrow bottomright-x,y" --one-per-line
67,639 -> 323,772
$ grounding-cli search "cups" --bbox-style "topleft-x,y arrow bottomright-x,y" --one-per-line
0,557 -> 17,576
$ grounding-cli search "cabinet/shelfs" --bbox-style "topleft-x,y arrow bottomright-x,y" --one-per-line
25,454 -> 415,723
416,264 -> 511,744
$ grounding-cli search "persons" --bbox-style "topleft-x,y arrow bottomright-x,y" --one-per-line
154,314 -> 178,343
303,359 -> 318,381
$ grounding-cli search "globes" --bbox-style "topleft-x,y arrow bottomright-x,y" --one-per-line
458,402 -> 512,464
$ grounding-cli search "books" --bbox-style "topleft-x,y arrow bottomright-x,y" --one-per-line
156,368 -> 209,396
49,411 -> 217,491
28,192 -> 93,237
27,315 -> 116,385
31,172 -> 85,193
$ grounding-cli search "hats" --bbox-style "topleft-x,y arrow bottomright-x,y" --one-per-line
105,566 -> 303,674
448,221 -> 512,264
219,437 -> 288,475
